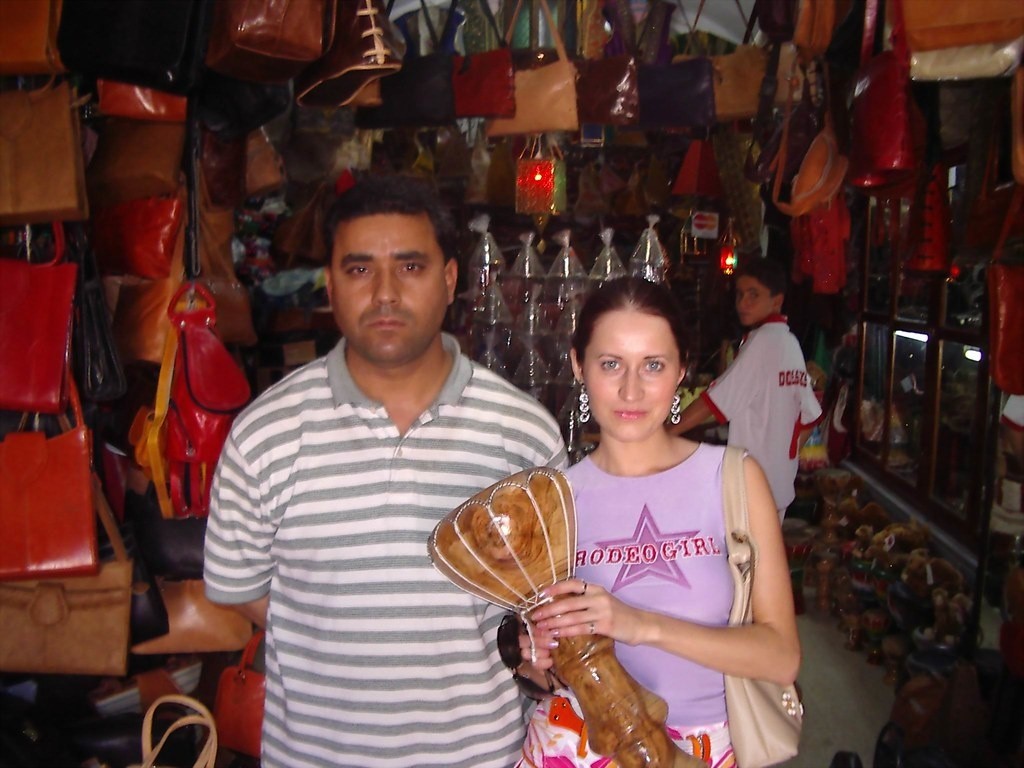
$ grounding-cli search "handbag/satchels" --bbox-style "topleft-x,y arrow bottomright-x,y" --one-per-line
0,0 -> 1024,757
720,445 -> 803,767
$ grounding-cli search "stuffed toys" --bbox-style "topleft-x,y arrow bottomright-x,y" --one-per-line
793,472 -> 975,640
890,662 -> 990,768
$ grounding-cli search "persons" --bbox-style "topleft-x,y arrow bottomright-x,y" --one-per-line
203,182 -> 569,768
666,267 -> 826,530
518,277 -> 801,768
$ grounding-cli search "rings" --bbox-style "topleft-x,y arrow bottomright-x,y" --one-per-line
578,581 -> 587,596
590,623 -> 594,634
